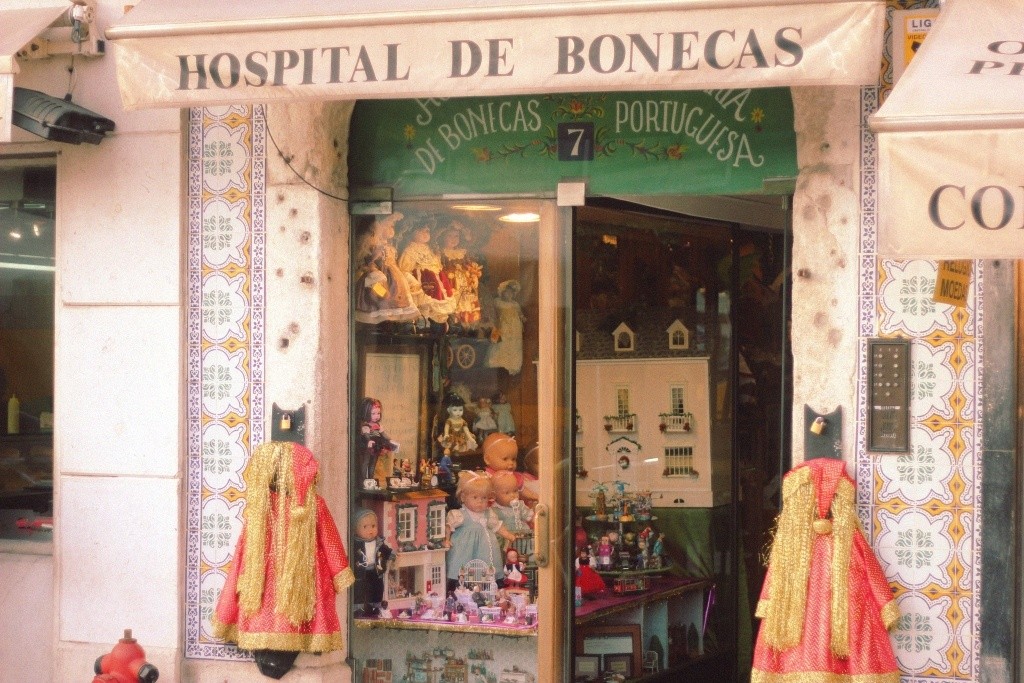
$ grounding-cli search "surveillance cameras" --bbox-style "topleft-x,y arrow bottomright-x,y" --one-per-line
12,86 -> 116,146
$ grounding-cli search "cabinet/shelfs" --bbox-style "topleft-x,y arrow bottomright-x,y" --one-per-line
350,578 -> 719,683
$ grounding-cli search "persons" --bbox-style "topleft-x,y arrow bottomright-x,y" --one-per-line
352,207 -> 529,375
349,390 -> 667,636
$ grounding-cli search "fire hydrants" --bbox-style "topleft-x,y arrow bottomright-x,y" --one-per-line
90,627 -> 160,683
